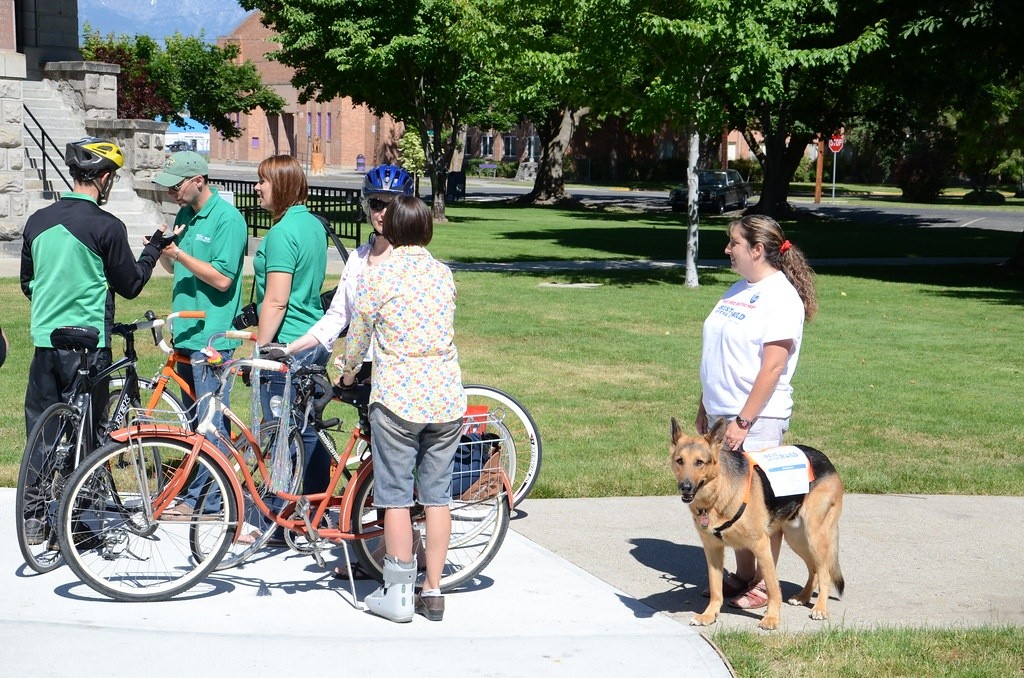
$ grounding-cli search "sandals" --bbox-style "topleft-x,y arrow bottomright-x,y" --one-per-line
332,560 -> 374,579
729,583 -> 781,608
700,571 -> 757,596
231,531 -> 293,546
159,504 -> 220,521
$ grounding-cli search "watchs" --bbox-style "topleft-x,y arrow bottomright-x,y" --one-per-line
735,415 -> 753,429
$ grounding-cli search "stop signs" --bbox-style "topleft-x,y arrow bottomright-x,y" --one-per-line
828,134 -> 843,153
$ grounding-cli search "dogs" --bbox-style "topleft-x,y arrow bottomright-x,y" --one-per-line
670,415 -> 845,630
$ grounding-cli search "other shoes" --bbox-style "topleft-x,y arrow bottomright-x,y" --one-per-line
22,518 -> 48,545
47,522 -> 106,550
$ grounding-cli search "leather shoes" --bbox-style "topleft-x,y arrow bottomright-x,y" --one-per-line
414,592 -> 445,621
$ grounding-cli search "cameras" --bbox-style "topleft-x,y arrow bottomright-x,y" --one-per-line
233,302 -> 259,330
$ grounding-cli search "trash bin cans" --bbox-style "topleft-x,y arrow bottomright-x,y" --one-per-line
446,170 -> 467,201
356,153 -> 366,171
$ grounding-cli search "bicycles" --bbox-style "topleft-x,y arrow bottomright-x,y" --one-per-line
15,308 -> 543,611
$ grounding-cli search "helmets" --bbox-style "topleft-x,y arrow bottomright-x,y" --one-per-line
361,165 -> 415,197
66,137 -> 124,180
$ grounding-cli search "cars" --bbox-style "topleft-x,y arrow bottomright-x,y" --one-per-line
668,168 -> 752,214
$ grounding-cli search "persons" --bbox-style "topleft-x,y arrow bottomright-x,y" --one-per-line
258,162 -> 419,583
232,154 -> 338,552
19,134 -> 187,551
696,206 -> 820,608
141,151 -> 247,524
334,190 -> 468,623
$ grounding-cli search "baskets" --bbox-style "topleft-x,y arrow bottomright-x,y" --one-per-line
451,412 -> 505,500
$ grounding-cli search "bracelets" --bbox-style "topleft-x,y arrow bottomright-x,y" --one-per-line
339,376 -> 359,390
173,247 -> 180,262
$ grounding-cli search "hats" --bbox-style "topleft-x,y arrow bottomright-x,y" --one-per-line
151,151 -> 208,188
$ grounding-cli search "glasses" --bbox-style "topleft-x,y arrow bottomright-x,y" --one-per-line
172,175 -> 200,191
367,199 -> 391,211
99,172 -> 121,183
